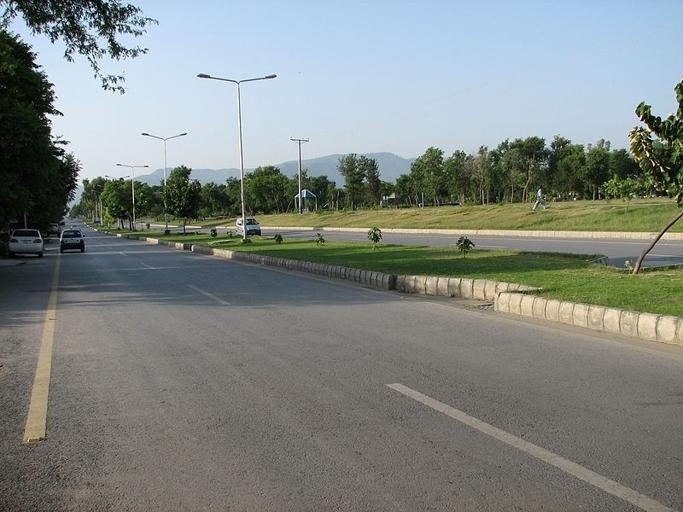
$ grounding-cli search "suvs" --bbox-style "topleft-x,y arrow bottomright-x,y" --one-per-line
7,220 -> 86,258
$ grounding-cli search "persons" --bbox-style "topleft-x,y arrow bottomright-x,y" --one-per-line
530,183 -> 546,211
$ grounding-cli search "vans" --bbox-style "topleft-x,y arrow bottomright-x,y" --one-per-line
234,216 -> 262,236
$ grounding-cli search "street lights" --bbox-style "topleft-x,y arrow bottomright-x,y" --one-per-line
105,175 -> 130,230
91,189 -> 103,227
140,131 -> 189,230
195,71 -> 279,242
115,163 -> 149,230
